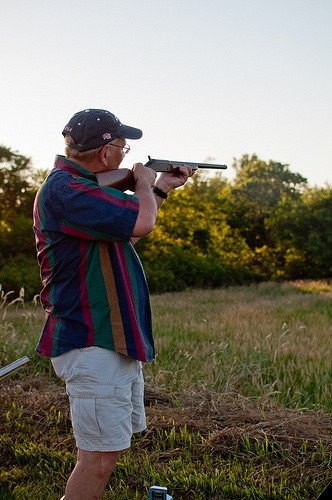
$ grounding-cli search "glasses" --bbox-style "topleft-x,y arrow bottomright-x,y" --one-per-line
108,143 -> 131,154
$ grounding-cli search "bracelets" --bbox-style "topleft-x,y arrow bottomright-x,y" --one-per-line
151,185 -> 168,200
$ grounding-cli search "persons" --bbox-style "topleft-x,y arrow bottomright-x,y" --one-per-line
30,107 -> 201,500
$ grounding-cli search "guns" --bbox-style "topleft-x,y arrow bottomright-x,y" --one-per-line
90,155 -> 227,193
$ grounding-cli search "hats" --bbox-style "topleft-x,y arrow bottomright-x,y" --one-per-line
62,109 -> 143,152
